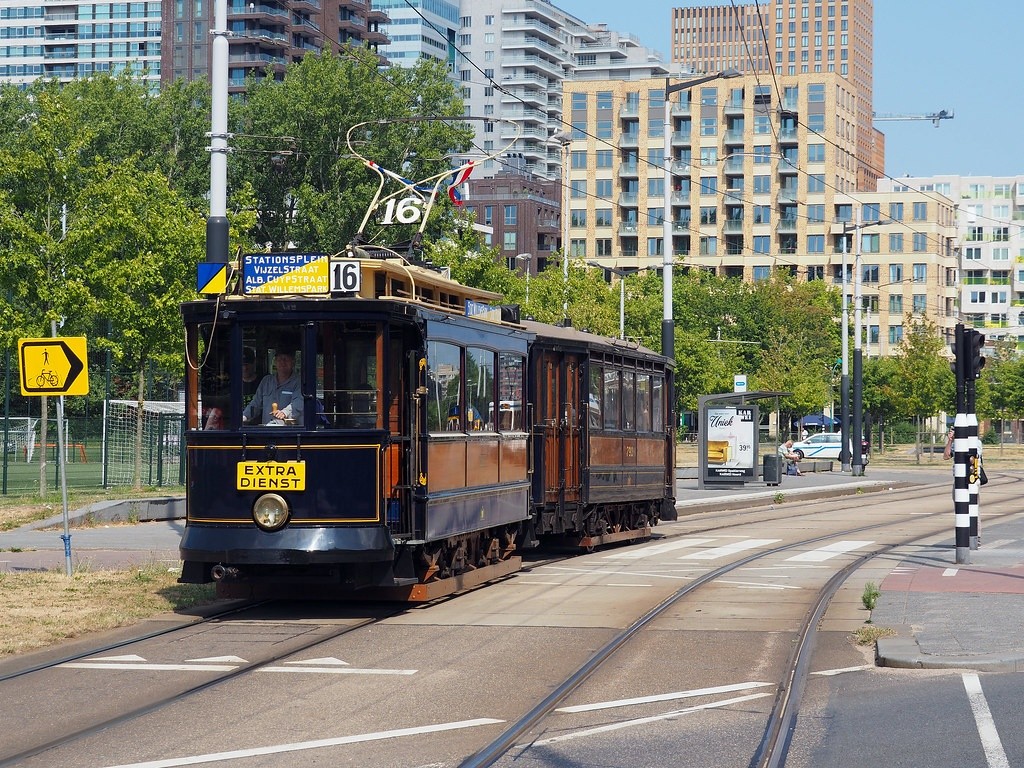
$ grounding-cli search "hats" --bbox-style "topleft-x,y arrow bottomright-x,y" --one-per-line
242,347 -> 255,365
274,348 -> 295,357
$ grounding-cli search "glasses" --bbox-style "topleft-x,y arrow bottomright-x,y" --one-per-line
276,357 -> 292,362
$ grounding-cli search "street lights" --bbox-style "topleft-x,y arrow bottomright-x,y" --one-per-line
516,252 -> 533,307
554,131 -> 573,319
660,69 -> 744,521
841,220 -> 892,477
587,261 -> 657,340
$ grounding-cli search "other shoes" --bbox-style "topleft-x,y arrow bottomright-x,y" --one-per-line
978,542 -> 981,548
797,472 -> 804,476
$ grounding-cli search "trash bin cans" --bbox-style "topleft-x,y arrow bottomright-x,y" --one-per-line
763,454 -> 782,486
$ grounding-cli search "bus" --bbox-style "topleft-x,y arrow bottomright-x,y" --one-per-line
177,116 -> 678,604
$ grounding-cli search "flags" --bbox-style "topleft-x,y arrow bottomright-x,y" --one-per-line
447,161 -> 475,206
370,161 -> 441,193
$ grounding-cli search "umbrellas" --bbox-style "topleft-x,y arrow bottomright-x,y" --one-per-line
793,412 -> 838,430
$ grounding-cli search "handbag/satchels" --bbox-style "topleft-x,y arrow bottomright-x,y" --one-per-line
980,466 -> 988,485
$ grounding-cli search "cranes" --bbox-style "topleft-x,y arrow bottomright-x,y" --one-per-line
873,108 -> 954,128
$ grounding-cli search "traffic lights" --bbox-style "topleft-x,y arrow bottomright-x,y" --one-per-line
951,324 -> 986,376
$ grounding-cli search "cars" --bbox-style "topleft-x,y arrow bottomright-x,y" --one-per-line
787,430 -> 853,464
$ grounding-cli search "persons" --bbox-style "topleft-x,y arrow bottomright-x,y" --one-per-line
943,426 -> 982,546
862,436 -> 869,445
802,428 -> 808,440
778,440 -> 805,476
340,384 -> 374,425
242,345 -> 304,428
23,445 -> 27,456
